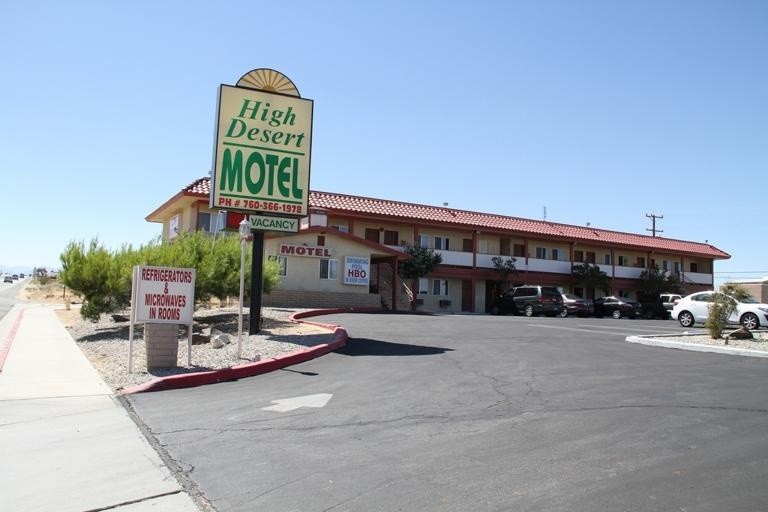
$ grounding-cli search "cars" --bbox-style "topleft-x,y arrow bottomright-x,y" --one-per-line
593,293 -> 687,319
560,293 -> 594,318
671,290 -> 768,330
3,274 -> 24,284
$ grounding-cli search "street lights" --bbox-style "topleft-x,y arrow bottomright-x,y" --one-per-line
238,215 -> 253,358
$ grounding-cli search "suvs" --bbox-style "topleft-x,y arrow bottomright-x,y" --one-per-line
489,284 -> 565,317
